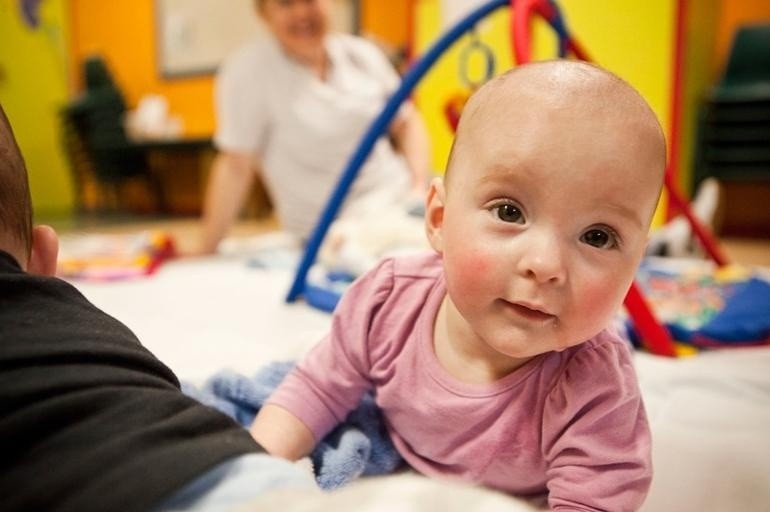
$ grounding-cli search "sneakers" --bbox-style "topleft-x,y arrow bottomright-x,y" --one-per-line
664,178 -> 720,259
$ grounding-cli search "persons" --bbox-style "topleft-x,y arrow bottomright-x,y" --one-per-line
173,0 -> 721,280
0,102 -> 533,512
252,59 -> 666,512
